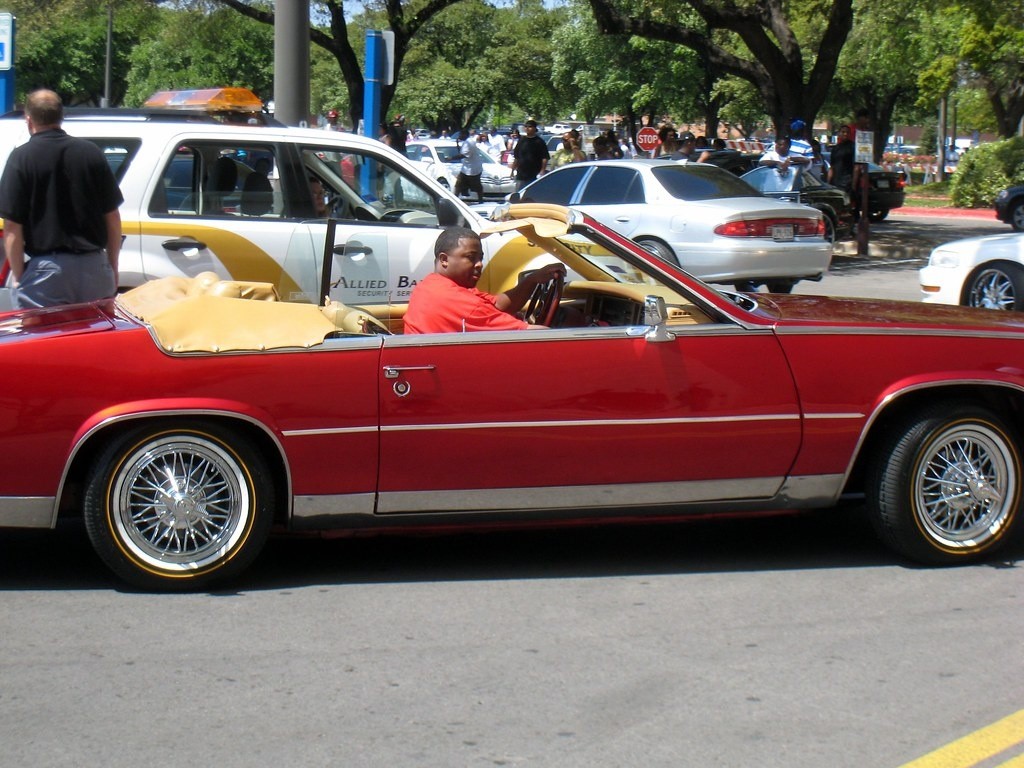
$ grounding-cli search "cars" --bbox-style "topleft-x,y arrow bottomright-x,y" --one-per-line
101,118 -> 977,240
918,233 -> 1024,313
993,186 -> 1024,233
470,157 -> 834,293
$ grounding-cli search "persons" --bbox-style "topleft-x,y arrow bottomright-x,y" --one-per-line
945,145 -> 969,165
551,129 -> 586,170
379,114 -> 419,202
760,120 -> 855,202
469,126 -> 520,167
510,120 -> 550,192
311,178 -> 326,215
446,129 -> 483,203
592,130 -> 632,160
652,128 -> 726,163
426,130 -> 451,139
325,111 -> 345,175
402,226 -> 566,335
0,89 -> 124,309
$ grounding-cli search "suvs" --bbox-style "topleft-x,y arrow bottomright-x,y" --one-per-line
0,87 -> 585,307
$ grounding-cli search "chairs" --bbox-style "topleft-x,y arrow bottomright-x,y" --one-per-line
319,298 -> 396,335
206,157 -> 237,212
240,171 -> 275,217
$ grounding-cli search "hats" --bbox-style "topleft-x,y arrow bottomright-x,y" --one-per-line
790,120 -> 807,130
676,131 -> 694,141
523,120 -> 536,126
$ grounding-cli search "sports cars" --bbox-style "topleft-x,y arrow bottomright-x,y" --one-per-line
0,199 -> 1024,595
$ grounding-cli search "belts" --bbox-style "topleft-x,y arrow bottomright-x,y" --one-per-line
31,248 -> 100,257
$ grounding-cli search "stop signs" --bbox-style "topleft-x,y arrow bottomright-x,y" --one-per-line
635,127 -> 659,153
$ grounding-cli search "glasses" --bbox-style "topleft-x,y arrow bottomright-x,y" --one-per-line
561,138 -> 569,142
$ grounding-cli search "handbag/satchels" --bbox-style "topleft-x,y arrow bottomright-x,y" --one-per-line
501,150 -> 515,162
